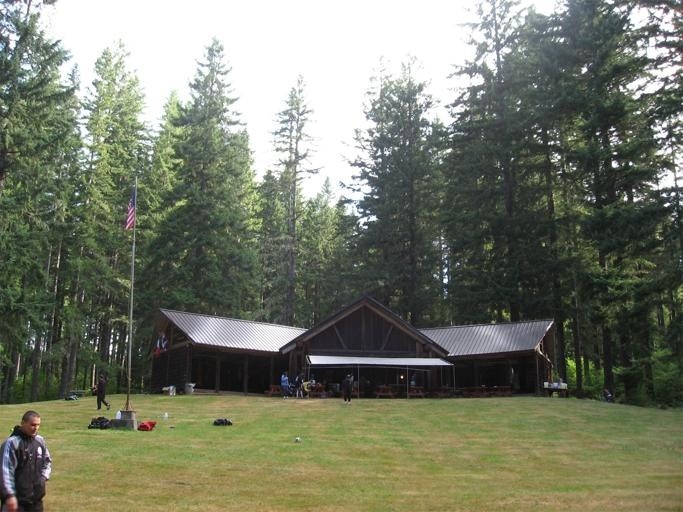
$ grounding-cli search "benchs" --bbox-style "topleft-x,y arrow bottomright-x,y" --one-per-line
262,381 -> 513,397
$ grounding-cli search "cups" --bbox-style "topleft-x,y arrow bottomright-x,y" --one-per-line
543,378 -> 567,389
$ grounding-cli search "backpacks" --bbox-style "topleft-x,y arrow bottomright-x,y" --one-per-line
214,418 -> 232,427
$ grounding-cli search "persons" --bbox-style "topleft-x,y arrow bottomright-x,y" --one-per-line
547,376 -> 554,397
293,373 -> 303,398
93,378 -> 109,410
0,410 -> 53,512
340,373 -> 353,404
302,380 -> 311,396
603,388 -> 615,403
279,371 -> 289,400
555,377 -> 563,393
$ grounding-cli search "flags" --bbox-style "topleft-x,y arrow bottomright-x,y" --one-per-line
125,186 -> 134,230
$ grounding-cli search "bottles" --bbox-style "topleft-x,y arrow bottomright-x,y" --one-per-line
162,412 -> 168,421
